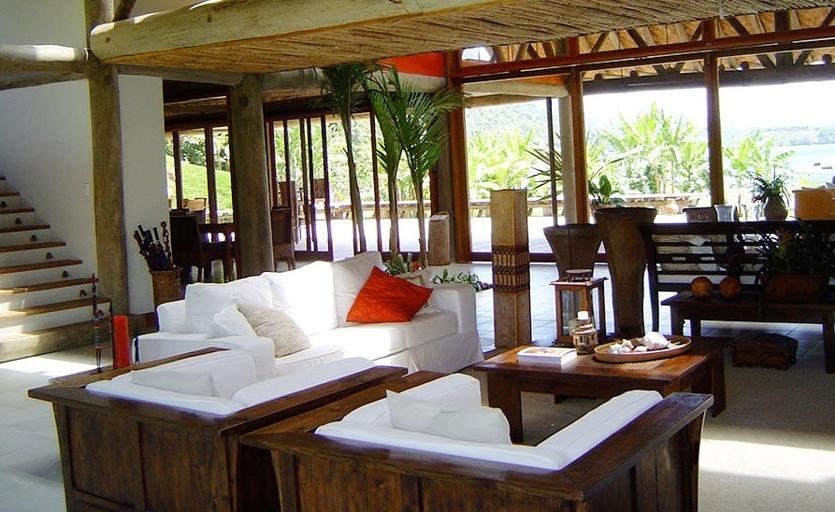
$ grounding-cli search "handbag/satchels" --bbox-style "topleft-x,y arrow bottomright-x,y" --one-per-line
734,333 -> 798,370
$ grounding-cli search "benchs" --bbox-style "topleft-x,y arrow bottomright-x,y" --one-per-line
638,219 -> 835,335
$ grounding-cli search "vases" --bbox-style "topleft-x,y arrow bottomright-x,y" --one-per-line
683,207 -> 716,223
545,223 -> 602,328
714,205 -> 733,222
594,207 -> 657,339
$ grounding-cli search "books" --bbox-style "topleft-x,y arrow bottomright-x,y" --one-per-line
516,347 -> 578,365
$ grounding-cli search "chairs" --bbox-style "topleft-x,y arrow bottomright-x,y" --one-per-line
170,216 -> 224,283
270,207 -> 294,272
186,210 -> 208,242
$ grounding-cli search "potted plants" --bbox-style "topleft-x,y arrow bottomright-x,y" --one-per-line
747,228 -> 835,299
746,169 -> 792,222
589,175 -> 626,220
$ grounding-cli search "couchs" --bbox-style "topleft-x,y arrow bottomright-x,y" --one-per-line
240,370 -> 715,512
132,251 -> 486,375
28,346 -> 408,511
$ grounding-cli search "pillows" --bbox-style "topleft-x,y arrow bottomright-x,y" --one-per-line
386,389 -> 513,446
259,261 -> 338,337
345,265 -> 434,323
208,308 -> 257,339
185,276 -> 272,333
237,303 -> 310,357
332,251 -> 383,328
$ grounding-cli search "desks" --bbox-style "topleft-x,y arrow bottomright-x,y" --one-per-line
550,276 -> 607,347
195,222 -> 237,281
